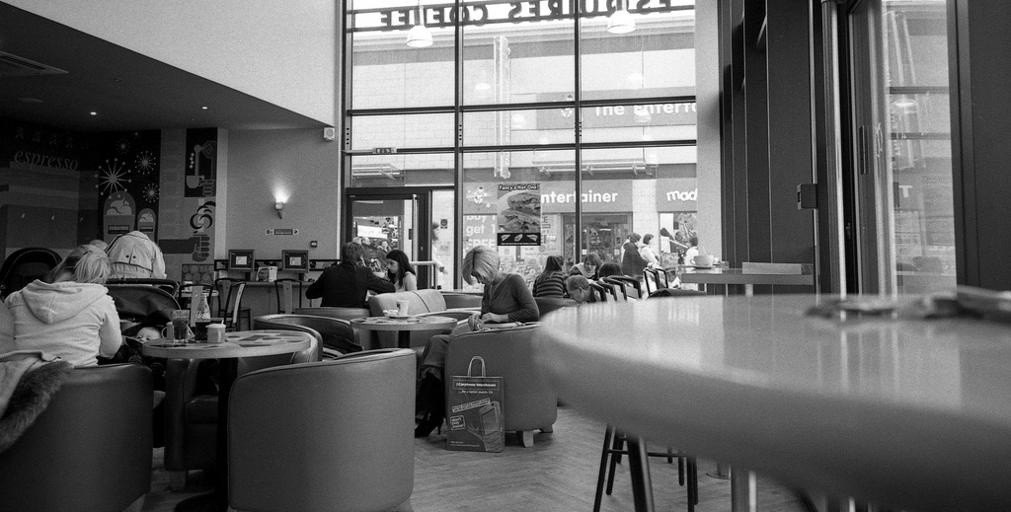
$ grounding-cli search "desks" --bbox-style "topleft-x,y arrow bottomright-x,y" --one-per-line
348,316 -> 458,349
679,266 -> 813,481
173,287 -> 221,298
527,293 -> 1011,512
142,328 -> 312,512
217,280 -> 315,307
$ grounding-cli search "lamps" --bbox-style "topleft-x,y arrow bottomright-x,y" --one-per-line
274,200 -> 287,213
405,23 -> 434,49
606,9 -> 636,36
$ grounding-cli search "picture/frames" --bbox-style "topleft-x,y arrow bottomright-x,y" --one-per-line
282,249 -> 309,273
228,248 -> 256,273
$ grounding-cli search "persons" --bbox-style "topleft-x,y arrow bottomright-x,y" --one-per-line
103,230 -> 167,278
431,224 -> 448,289
621,227 -> 699,275
0,243 -> 123,370
565,275 -> 593,304
414,244 -> 540,438
107,325 -> 162,369
569,251 -> 602,276
305,241 -> 396,308
532,255 -> 567,298
350,235 -> 391,270
382,250 -> 417,293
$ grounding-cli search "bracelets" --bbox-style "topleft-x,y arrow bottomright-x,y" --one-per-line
439,266 -> 444,272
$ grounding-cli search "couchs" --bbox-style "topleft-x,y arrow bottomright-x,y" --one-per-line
0,363 -> 156,512
294,307 -> 370,320
444,321 -> 558,447
227,347 -> 417,511
368,288 -> 483,355
253,313 -> 366,359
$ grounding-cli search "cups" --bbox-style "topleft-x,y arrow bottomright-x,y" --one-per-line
689,253 -> 715,269
396,299 -> 410,317
172,309 -> 190,347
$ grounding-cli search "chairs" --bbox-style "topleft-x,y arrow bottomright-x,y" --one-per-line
223,280 -> 247,332
609,272 -> 694,486
210,278 -> 241,325
180,283 -> 214,317
275,278 -> 302,313
594,276 -> 700,511
643,267 -> 705,297
654,266 -> 669,288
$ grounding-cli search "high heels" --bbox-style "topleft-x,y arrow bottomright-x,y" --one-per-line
415,409 -> 445,440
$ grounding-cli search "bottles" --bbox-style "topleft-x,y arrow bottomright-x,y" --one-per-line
195,293 -> 210,342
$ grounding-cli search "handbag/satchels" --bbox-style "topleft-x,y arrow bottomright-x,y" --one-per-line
446,356 -> 508,453
